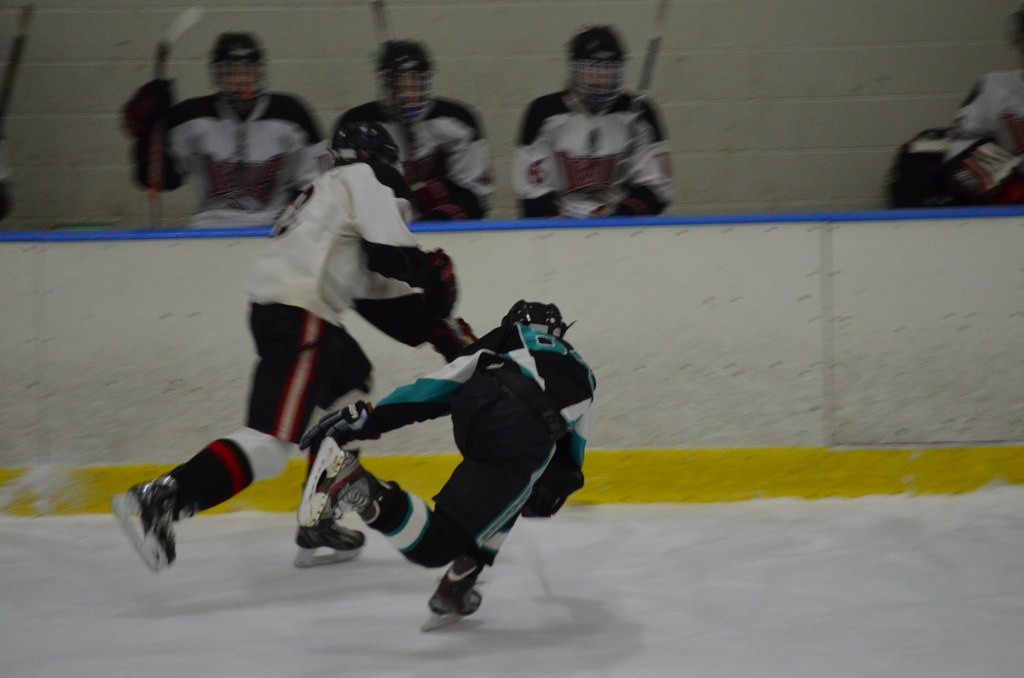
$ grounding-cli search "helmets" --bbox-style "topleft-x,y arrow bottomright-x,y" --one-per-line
507,298 -> 562,330
332,122 -> 401,166
567,28 -> 628,105
374,39 -> 435,112
209,31 -> 267,95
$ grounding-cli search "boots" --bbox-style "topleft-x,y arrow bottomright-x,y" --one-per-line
111,472 -> 197,566
298,438 -> 374,529
419,559 -> 484,631
293,502 -> 363,569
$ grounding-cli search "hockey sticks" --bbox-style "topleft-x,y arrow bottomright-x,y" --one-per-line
605,0 -> 670,219
367,0 -> 433,223
149,3 -> 204,231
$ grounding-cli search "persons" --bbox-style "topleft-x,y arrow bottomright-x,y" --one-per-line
331,39 -> 497,221
111,120 -> 457,575
298,296 -> 598,630
942,2 -> 1024,206
511,24 -> 675,218
121,30 -> 324,229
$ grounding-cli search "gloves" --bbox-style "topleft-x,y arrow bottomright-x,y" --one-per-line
427,321 -> 476,360
300,399 -> 375,452
426,249 -> 458,319
